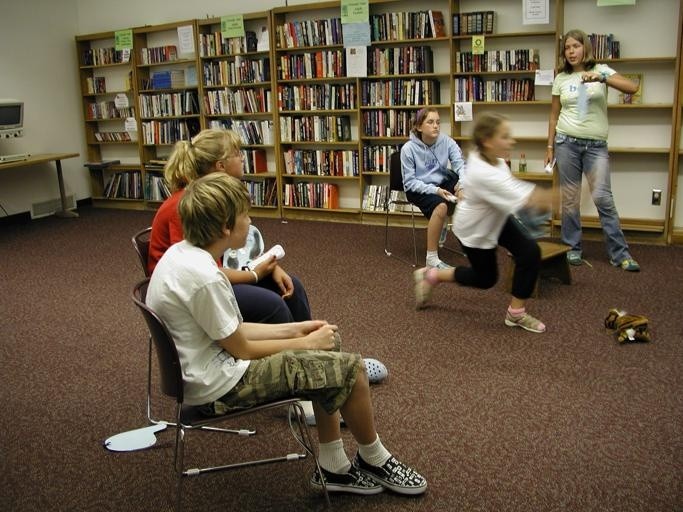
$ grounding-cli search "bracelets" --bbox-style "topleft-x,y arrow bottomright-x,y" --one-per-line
250,271 -> 259,283
600,73 -> 606,83
547,145 -> 553,148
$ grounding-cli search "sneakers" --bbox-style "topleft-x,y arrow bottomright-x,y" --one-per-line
504,307 -> 547,334
425,256 -> 456,271
308,461 -> 387,495
621,258 -> 641,272
412,266 -> 435,310
351,448 -> 429,495
566,249 -> 584,266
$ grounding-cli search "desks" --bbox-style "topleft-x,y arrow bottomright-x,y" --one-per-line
0,152 -> 80,219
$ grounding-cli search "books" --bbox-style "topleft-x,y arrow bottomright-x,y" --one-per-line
362,10 -> 446,214
199,26 -> 277,206
87,46 -> 143,199
618,69 -> 643,104
455,11 -> 537,101
276,18 -> 359,208
558,33 -> 620,64
138,46 -> 200,200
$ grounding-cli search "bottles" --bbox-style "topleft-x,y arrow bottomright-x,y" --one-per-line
519,153 -> 527,173
505,153 -> 511,172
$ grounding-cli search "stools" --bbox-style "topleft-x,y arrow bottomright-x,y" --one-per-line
506,242 -> 573,298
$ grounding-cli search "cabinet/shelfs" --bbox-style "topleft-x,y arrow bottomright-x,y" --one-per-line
196,10 -> 281,219
133,19 -> 204,212
271,1 -> 361,224
75,26 -> 147,211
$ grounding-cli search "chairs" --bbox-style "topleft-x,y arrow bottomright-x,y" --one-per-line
131,277 -> 339,511
131,226 -> 259,436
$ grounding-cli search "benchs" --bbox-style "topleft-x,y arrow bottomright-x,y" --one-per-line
384,151 -> 469,267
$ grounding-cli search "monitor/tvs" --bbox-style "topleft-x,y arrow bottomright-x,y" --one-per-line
0,99 -> 24,130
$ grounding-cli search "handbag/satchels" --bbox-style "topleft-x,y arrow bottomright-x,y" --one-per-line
445,168 -> 459,184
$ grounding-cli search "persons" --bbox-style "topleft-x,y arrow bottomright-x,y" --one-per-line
543,30 -> 642,272
144,171 -> 427,496
147,128 -> 386,425
400,107 -> 466,269
411,113 -> 546,334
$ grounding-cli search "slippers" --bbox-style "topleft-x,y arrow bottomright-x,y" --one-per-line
298,407 -> 346,428
362,358 -> 387,382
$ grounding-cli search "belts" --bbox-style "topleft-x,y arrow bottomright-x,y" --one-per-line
555,133 -> 607,146
